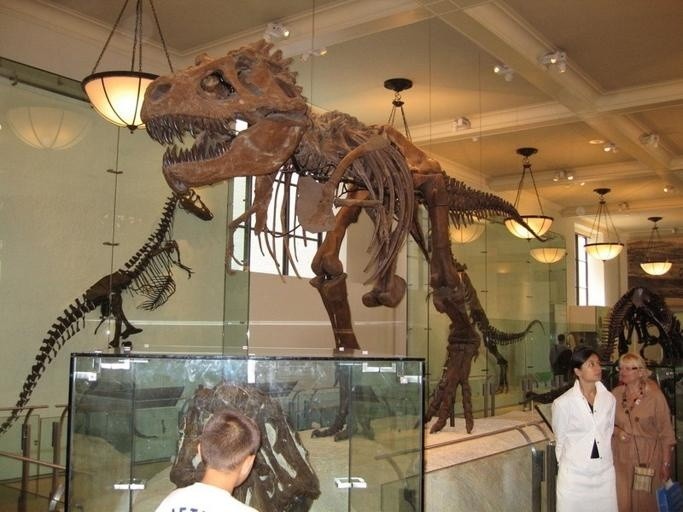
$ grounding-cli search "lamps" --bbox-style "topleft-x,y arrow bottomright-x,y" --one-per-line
503,147 -> 673,277
81,1 -> 176,133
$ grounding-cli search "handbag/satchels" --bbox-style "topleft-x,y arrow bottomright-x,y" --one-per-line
631,465 -> 655,493
655,476 -> 683,512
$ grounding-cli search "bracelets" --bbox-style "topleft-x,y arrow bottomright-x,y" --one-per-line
661,461 -> 670,467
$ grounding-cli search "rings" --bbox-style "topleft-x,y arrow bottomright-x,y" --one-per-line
621,435 -> 625,440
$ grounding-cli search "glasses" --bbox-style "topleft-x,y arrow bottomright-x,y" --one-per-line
615,366 -> 643,372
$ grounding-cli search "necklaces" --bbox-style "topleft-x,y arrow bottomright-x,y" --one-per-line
621,379 -> 647,413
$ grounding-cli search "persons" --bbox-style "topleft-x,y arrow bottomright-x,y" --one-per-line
548,333 -> 572,389
609,351 -> 678,512
150,409 -> 263,511
550,347 -> 619,511
